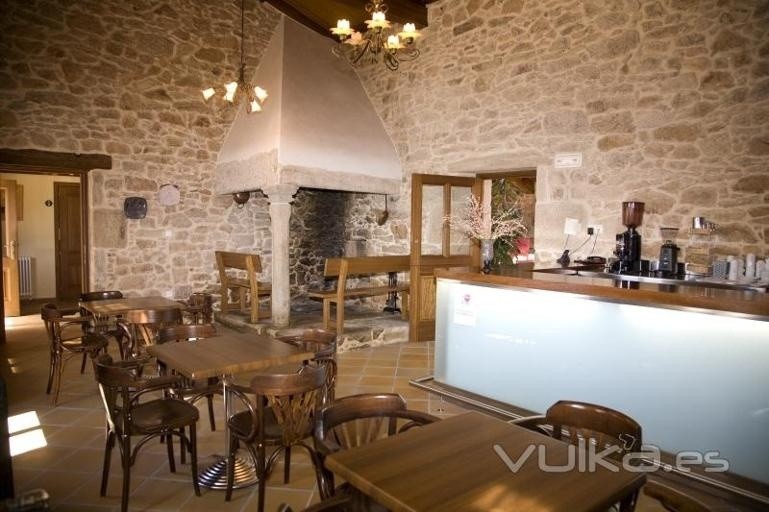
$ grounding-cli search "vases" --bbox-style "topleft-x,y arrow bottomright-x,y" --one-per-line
479,239 -> 496,271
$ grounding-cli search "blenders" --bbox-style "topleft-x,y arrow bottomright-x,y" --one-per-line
659,227 -> 679,275
607,200 -> 645,274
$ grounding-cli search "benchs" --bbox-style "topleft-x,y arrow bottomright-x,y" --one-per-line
215,251 -> 271,323
308,255 -> 411,334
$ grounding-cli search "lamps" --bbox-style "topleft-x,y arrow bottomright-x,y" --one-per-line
330,0 -> 422,71
201,0 -> 269,114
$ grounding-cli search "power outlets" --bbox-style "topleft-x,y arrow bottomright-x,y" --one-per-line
586,225 -> 602,237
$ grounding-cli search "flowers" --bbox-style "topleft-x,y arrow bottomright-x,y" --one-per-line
440,192 -> 528,259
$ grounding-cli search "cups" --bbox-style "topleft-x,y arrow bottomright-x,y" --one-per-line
692,217 -> 704,229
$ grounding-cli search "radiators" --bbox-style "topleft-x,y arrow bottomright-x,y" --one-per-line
19,256 -> 32,301
